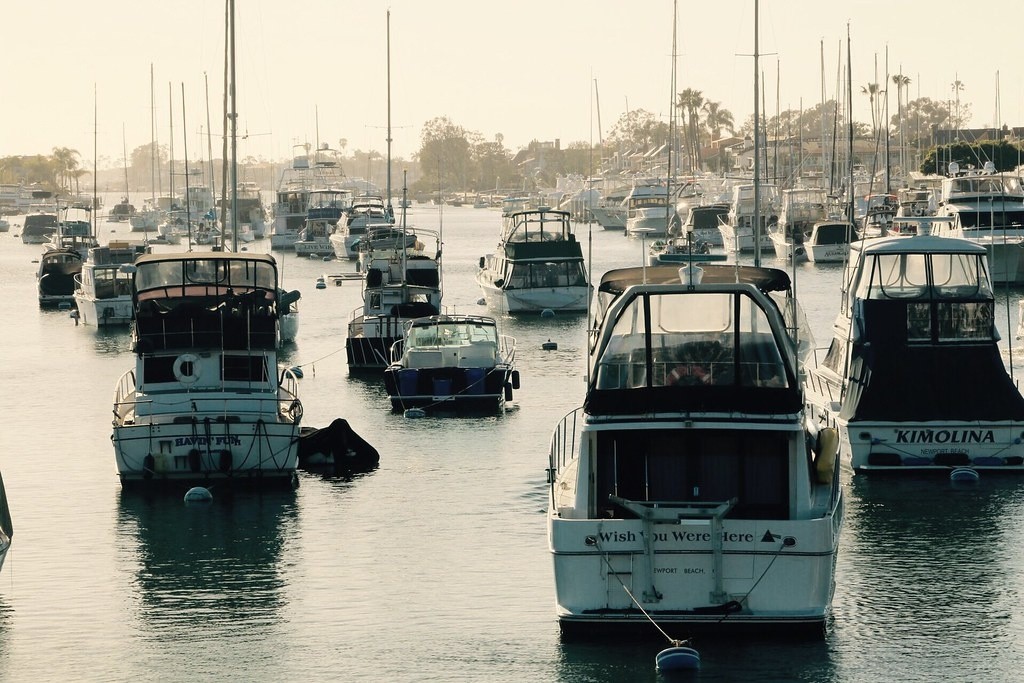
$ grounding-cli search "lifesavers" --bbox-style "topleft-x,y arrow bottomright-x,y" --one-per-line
172,354 -> 204,384
914,208 -> 923,216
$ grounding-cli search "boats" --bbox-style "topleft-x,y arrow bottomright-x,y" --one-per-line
931,162 -> 1024,284
109,0 -> 303,488
42,202 -> 100,251
888,183 -> 941,237
21,213 -> 57,242
328,194 -> 395,261
416,187 -> 524,208
294,143 -> 347,256
551,0 -> 858,261
383,314 -> 517,415
130,60 -> 266,244
546,0 -> 844,623
109,196 -> 136,222
267,143 -> 313,250
804,217 -> 1024,472
858,196 -> 898,240
70,239 -> 154,328
35,247 -> 86,307
344,171 -> 445,375
355,223 -> 425,273
475,206 -> 594,314
